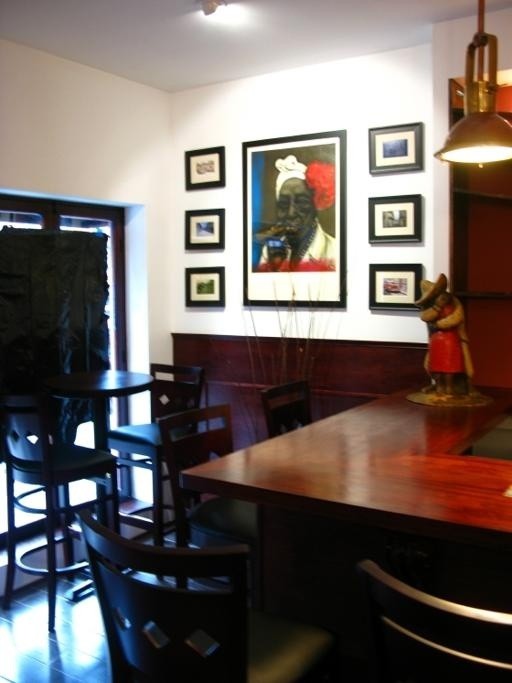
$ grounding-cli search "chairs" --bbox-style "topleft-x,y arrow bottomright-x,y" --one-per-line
109,363 -> 207,583
155,405 -> 317,615
363,558 -> 512,681
262,381 -> 380,580
0,406 -> 126,632
78,508 -> 340,683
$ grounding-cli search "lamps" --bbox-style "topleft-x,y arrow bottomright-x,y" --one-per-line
200,0 -> 223,15
434,1 -> 512,163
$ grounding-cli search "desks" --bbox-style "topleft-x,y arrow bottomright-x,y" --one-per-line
36,370 -> 189,598
180,381 -> 512,624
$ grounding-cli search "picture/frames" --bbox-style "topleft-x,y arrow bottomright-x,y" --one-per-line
184,208 -> 225,250
369,263 -> 425,312
242,129 -> 347,309
368,122 -> 425,175
368,193 -> 423,244
185,266 -> 226,308
184,145 -> 227,191
